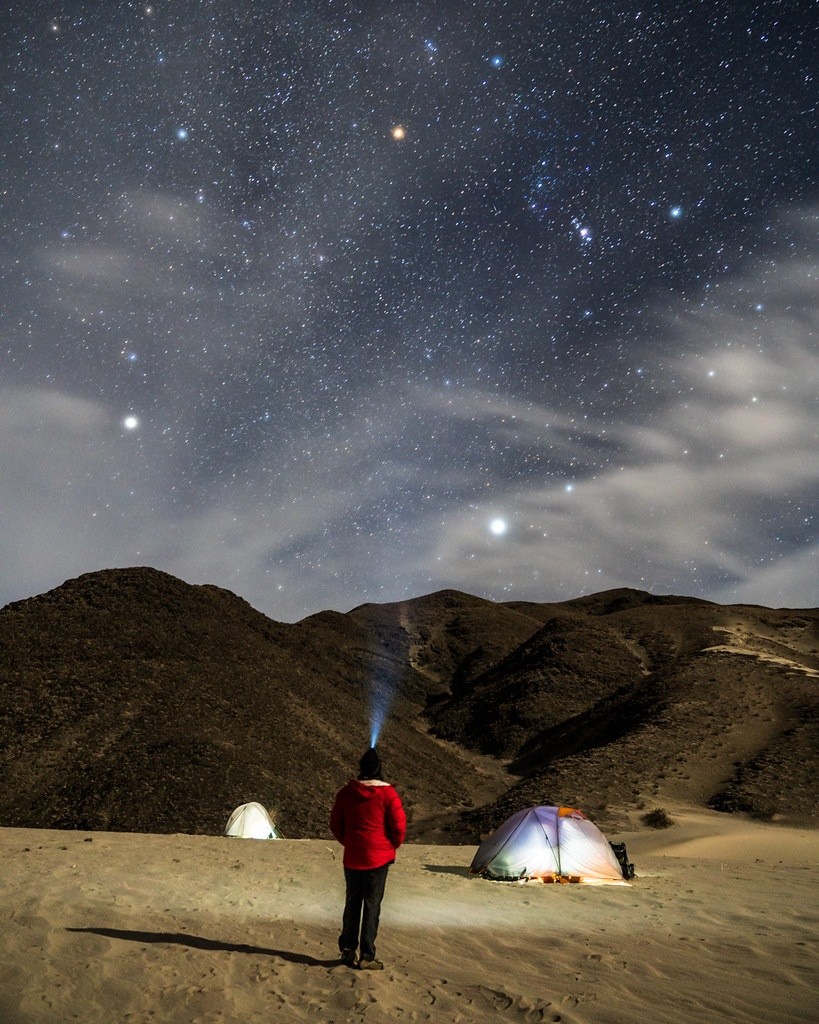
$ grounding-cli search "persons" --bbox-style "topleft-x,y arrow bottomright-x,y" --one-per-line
328,748 -> 406,970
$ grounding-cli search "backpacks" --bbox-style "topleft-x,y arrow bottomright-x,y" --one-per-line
609,841 -> 638,881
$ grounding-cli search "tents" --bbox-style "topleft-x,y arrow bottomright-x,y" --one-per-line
222,802 -> 286,840
467,806 -> 623,881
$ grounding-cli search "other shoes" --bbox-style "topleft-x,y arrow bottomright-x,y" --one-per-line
356,959 -> 383,970
341,948 -> 357,965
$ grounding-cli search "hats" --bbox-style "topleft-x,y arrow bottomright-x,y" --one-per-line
359,748 -> 382,778
